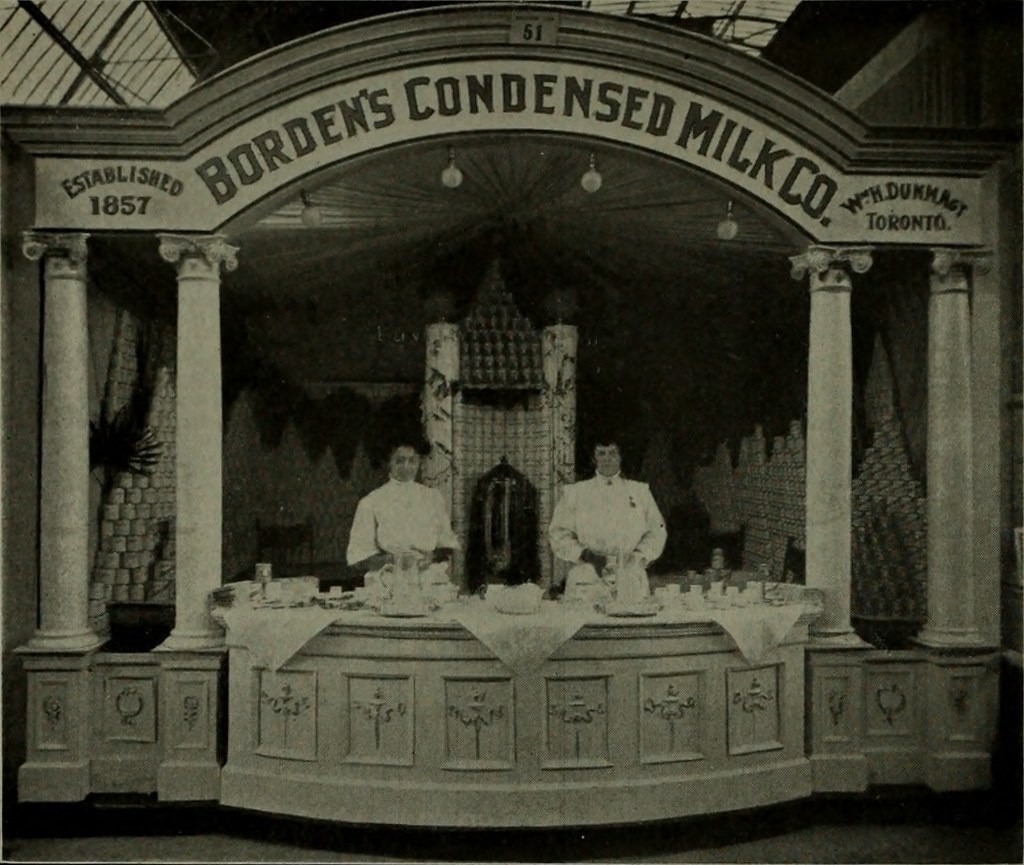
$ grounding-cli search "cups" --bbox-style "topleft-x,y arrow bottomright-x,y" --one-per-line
655,588 -> 666,602
486,582 -> 505,607
685,584 -> 704,609
746,582 -> 765,603
708,582 -> 722,603
247,562 -> 281,608
726,585 -> 738,604
665,584 -> 681,597
329,586 -> 342,595
354,588 -> 367,598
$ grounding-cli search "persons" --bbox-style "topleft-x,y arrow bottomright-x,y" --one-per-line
547,433 -> 669,593
341,434 -> 461,588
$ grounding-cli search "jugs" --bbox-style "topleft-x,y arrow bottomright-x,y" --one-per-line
380,550 -> 424,611
